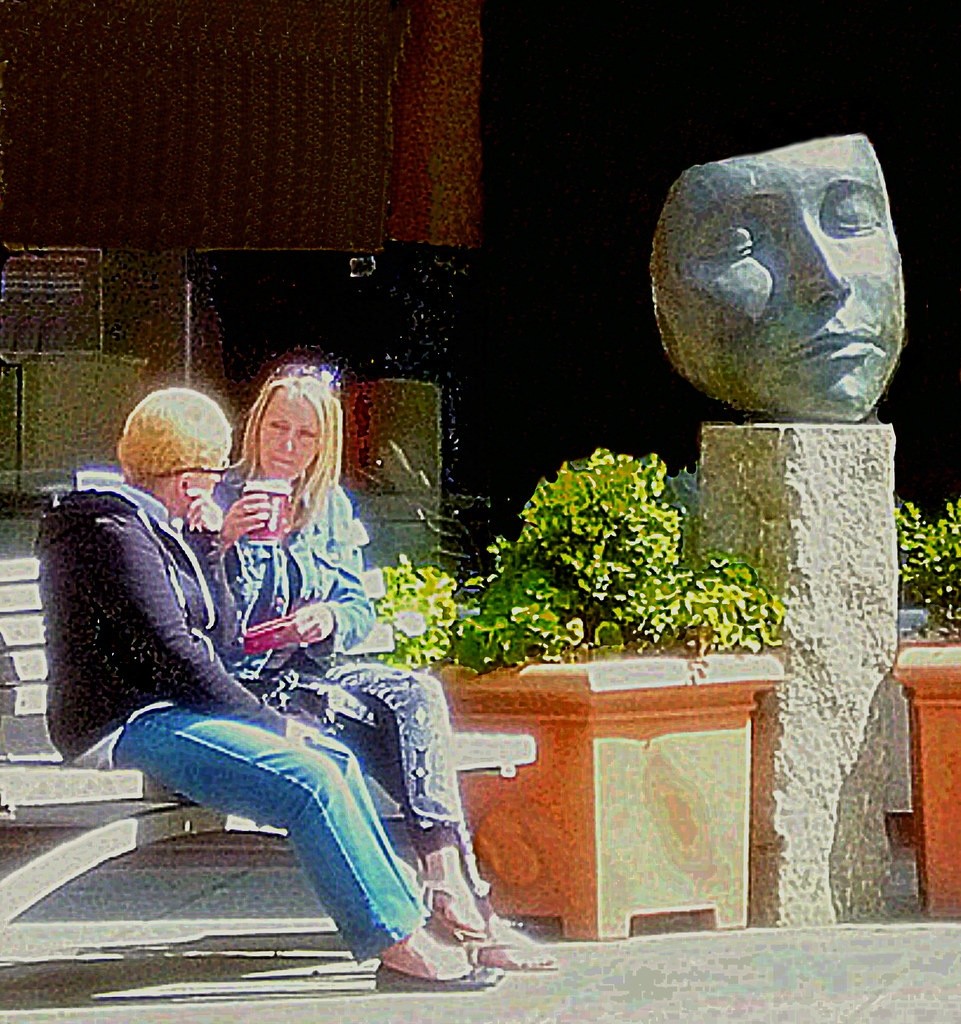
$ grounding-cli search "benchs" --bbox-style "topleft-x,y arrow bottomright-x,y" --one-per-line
1,554 -> 536,965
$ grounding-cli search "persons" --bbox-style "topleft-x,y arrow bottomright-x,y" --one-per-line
649,130 -> 908,422
215,365 -> 561,970
33,388 -> 504,992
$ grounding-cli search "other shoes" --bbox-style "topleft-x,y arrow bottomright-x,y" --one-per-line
375,962 -> 505,993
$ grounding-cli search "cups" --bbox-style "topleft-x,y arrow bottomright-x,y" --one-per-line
242,486 -> 291,547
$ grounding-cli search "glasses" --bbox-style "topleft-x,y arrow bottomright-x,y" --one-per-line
156,459 -> 229,487
268,363 -> 324,384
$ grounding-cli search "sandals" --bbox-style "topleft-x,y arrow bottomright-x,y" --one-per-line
421,879 -> 489,942
459,929 -> 559,972
